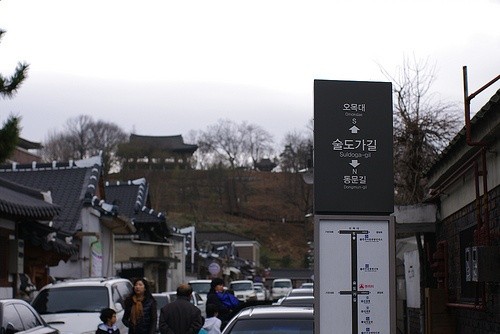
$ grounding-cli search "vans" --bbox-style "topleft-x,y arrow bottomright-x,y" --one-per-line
226,280 -> 257,305
153,291 -> 206,329
187,280 -> 212,303
272,278 -> 293,299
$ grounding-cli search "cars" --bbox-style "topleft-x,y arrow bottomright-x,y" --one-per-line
0,299 -> 60,334
220,306 -> 314,334
289,288 -> 314,297
300,283 -> 313,288
253,283 -> 270,303
272,297 -> 314,306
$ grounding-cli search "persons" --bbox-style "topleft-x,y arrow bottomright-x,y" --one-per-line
197,316 -> 208,334
159,283 -> 203,334
122,279 -> 157,334
203,303 -> 222,334
206,278 -> 235,332
95,308 -> 120,334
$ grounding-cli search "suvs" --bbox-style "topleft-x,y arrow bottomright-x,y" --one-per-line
29,276 -> 135,334
